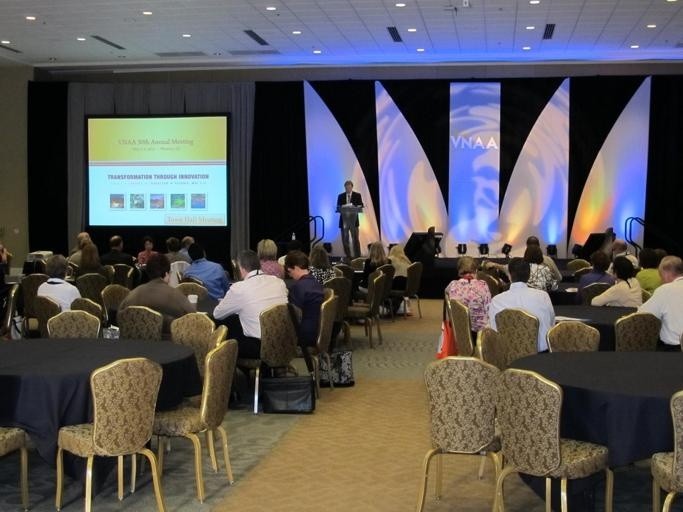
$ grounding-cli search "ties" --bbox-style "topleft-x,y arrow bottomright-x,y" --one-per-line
347,195 -> 351,202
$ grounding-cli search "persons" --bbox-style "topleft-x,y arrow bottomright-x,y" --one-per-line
1,246 -> 13,281
101,235 -> 141,279
579,251 -> 615,294
164,237 -> 191,265
137,237 -> 158,264
285,250 -> 324,348
490,257 -> 555,354
257,239 -> 285,280
448,255 -> 492,346
606,239 -> 638,274
214,250 -> 289,357
308,244 -> 336,284
637,255 -> 683,352
179,236 -> 196,254
117,254 -> 197,334
37,254 -> 81,313
636,249 -> 663,293
387,243 -> 413,317
70,232 -> 89,254
183,243 -> 230,298
358,241 -> 391,293
76,245 -> 110,283
591,256 -> 643,307
336,181 -> 364,258
486,246 -> 559,292
526,236 -> 562,280
71,239 -> 93,264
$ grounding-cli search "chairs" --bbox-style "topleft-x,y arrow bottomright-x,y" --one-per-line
579,283 -> 611,305
131,339 -> 235,504
478,272 -> 500,297
375,264 -> 396,323
642,289 -> 652,303
614,313 -> 662,351
171,312 -> 215,377
70,298 -> 119,339
445,295 -> 457,344
476,327 -> 509,370
495,308 -> 539,363
546,320 -> 600,352
168,261 -> 191,288
178,277 -> 205,288
391,262 -> 423,320
416,356 -> 501,512
450,299 -> 474,357
101,284 -> 132,326
651,390 -> 683,512
323,277 -> 352,348
21,274 -> 51,338
353,270 -> 383,337
117,306 -> 163,341
113,264 -> 133,285
104,265 -> 119,284
54,357 -> 166,512
127,268 -> 137,291
334,264 -> 354,281
271,289 -> 339,399
575,267 -> 592,282
23,296 -> 61,338
47,309 -> 101,339
478,261 -> 511,293
567,259 -> 590,271
174,283 -> 208,303
0,284 -> 19,337
76,273 -> 109,306
493,369 -> 613,512
348,274 -> 387,349
333,267 -> 343,277
236,304 -> 296,415
0,427 -> 31,512
179,325 -> 227,408
351,258 -> 368,270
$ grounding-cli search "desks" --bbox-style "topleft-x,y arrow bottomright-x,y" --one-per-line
354,269 -> 364,276
0,338 -> 202,502
108,298 -> 222,331
561,270 -> 580,283
553,305 -> 638,352
507,351 -> 683,512
547,283 -> 581,306
5,274 -> 75,286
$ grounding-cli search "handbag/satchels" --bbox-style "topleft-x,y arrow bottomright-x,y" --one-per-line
260,364 -> 317,415
11,317 -> 23,339
436,301 -> 458,360
317,350 -> 355,388
